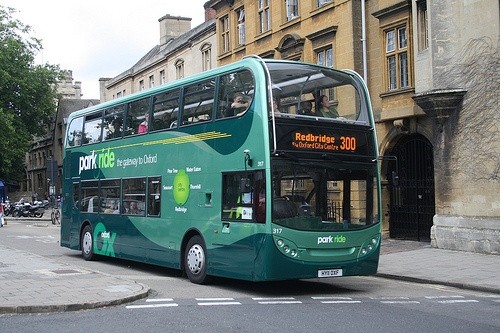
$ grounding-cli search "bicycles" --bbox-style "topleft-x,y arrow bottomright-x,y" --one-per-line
50,206 -> 61,225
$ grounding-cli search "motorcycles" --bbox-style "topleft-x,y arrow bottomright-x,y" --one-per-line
3,196 -> 49,218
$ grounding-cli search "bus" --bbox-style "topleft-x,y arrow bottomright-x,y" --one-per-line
60,55 -> 400,285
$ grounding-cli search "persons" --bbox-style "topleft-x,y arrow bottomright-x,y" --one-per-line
105,201 -> 126,214
128,201 -> 142,214
0,203 -> 3,227
269,98 -> 279,112
45,192 -> 62,208
317,95 -> 339,118
231,92 -> 248,115
158,112 -> 171,129
257,183 -> 289,220
298,101 -> 315,115
149,206 -> 159,215
138,112 -> 154,134
